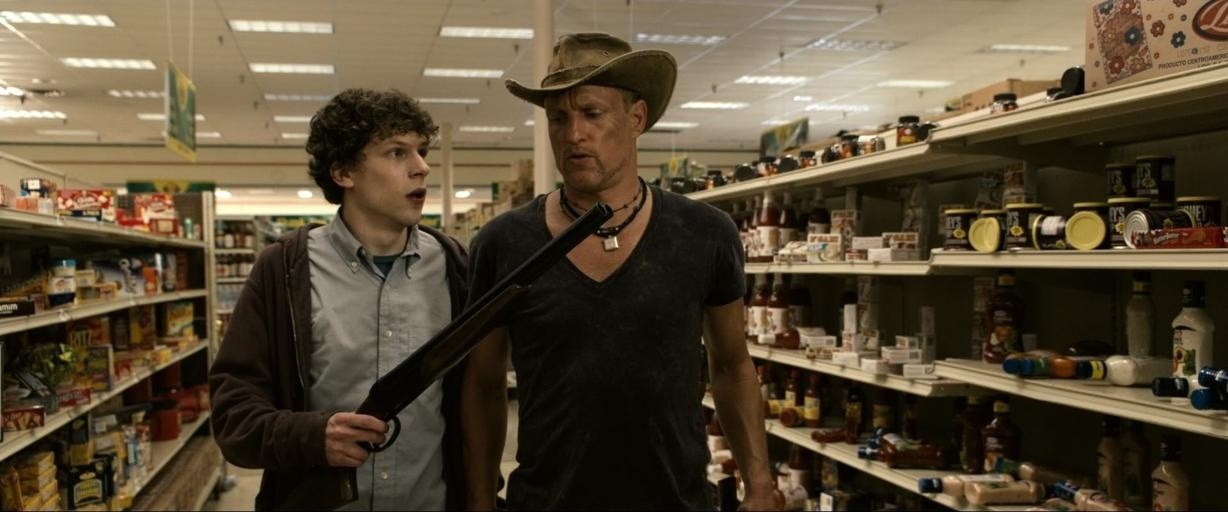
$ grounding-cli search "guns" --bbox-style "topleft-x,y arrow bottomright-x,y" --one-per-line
253,201 -> 613,512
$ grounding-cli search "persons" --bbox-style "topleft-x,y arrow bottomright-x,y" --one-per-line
444,28 -> 783,511
203,86 -> 505,510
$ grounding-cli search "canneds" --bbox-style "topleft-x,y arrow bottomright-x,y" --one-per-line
992,94 -> 1018,112
180,217 -> 194,240
942,155 -> 1224,251
670,115 -> 940,194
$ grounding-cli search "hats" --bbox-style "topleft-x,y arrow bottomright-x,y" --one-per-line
504,31 -> 681,129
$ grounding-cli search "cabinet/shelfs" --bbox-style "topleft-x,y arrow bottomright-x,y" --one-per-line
681,61 -> 1228,512
0,189 -> 254,512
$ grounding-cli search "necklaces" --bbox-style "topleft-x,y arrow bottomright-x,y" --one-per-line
556,176 -> 648,255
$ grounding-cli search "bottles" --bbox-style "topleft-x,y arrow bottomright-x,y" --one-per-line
754,360 -> 1192,511
1125,270 -> 1156,357
984,274 -> 1019,365
1170,277 -> 1217,399
1150,364 -> 1228,413
1074,353 -> 1172,389
747,274 -> 832,351
214,219 -> 256,310
1002,348 -> 1109,381
704,186 -> 860,264
